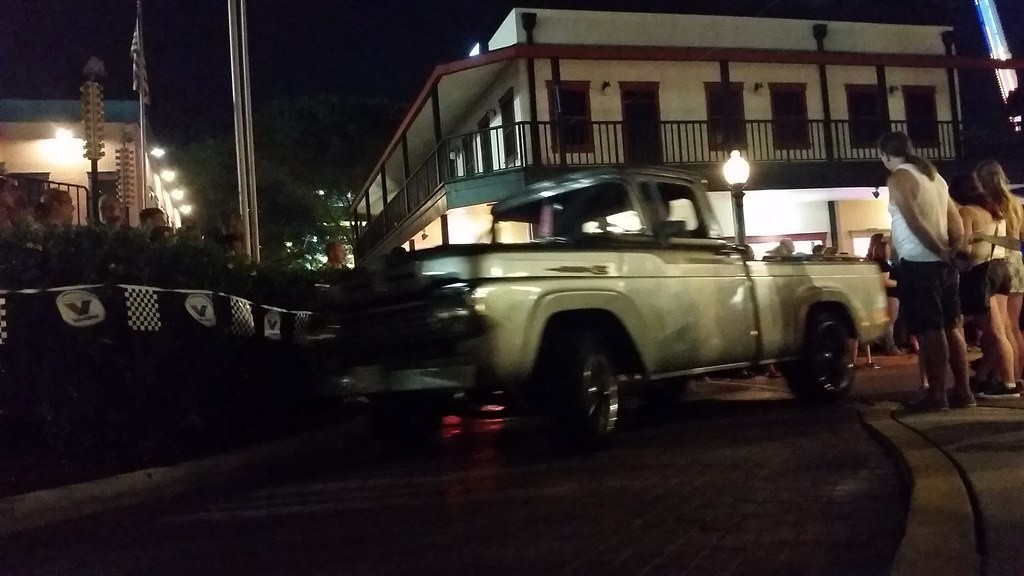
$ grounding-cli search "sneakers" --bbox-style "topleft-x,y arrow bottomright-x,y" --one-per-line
977,382 -> 1021,398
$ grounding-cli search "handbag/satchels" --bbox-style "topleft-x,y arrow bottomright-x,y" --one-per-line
960,261 -> 991,315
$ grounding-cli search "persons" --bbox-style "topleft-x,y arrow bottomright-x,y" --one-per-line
0,171 -> 350,273
878,132 -> 976,413
730,159 -> 1024,400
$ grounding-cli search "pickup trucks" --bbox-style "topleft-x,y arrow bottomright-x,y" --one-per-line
360,166 -> 890,440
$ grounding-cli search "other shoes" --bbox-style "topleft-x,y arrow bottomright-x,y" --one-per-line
866,363 -> 880,369
949,393 -> 977,407
902,393 -> 949,411
887,346 -> 905,357
847,361 -> 860,367
